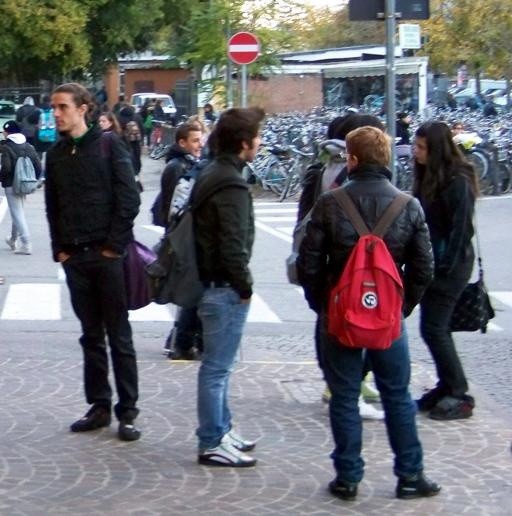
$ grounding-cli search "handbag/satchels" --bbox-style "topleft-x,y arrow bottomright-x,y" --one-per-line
449,279 -> 496,333
124,238 -> 156,311
151,188 -> 169,228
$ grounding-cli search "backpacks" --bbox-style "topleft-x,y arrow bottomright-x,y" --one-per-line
123,116 -> 142,143
144,170 -> 249,310
325,183 -> 411,350
3,140 -> 39,196
284,162 -> 350,287
21,106 -> 40,139
162,174 -> 197,222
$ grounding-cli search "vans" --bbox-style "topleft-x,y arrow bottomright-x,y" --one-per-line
128,92 -> 177,121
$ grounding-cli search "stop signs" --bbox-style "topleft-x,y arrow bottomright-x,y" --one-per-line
227,32 -> 260,66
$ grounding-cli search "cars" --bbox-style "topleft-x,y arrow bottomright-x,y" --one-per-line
434,85 -> 512,106
0,98 -> 19,135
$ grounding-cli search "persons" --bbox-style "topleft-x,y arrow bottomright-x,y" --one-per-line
296,125 -> 441,501
411,121 -> 481,421
396,112 -> 411,145
450,124 -> 464,137
295,113 -> 386,421
195,106 -> 265,467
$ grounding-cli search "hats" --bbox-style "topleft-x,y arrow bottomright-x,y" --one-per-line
3,120 -> 23,133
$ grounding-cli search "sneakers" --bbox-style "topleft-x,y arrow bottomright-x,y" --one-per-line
162,341 -> 205,362
197,442 -> 257,468
215,429 -> 256,452
327,477 -> 358,501
394,475 -> 443,501
356,396 -> 386,420
358,378 -> 382,401
429,396 -> 474,419
322,381 -> 334,402
71,403 -> 112,433
413,387 -> 448,412
117,421 -> 141,442
5,235 -> 34,256
